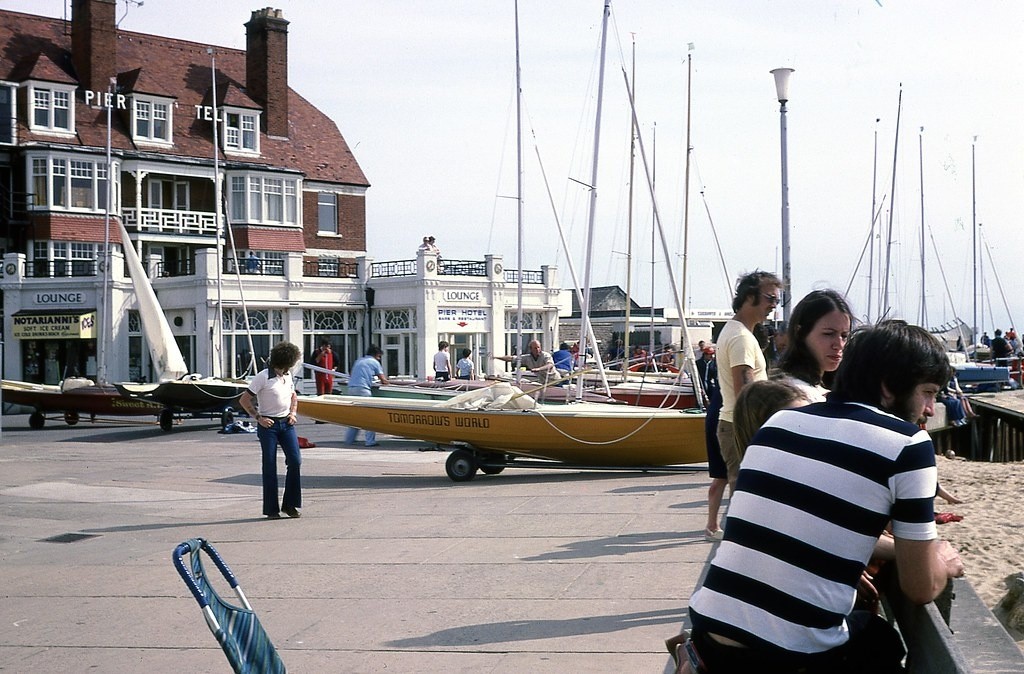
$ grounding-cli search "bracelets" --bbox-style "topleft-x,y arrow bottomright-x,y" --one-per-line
292,412 -> 297,418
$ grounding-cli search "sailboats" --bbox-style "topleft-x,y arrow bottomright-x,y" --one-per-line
0,83 -> 186,415
298,0 -> 1024,466
112,59 -> 258,411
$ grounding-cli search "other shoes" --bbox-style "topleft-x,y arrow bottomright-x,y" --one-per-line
281,503 -> 301,517
268,514 -> 280,519
365,443 -> 380,447
949,411 -> 980,427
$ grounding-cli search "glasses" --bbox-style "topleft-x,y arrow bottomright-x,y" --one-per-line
758,290 -> 780,304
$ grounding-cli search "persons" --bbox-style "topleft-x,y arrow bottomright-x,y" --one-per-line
245,250 -> 260,273
980,328 -> 1019,369
63,360 -> 78,379
606,339 -> 648,371
239,342 -> 302,519
554,342 -> 580,376
936,366 -> 979,427
311,337 -> 339,394
455,348 -> 474,380
433,341 -> 452,381
495,340 -> 561,387
715,271 -> 781,500
665,289 -> 964,674
346,345 -> 387,446
419,236 -> 441,258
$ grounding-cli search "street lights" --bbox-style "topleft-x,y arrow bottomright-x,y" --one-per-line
769,68 -> 798,338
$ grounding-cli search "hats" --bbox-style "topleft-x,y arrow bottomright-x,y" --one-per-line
703,346 -> 714,354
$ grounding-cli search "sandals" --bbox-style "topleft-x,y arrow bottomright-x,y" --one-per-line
705,528 -> 725,542
